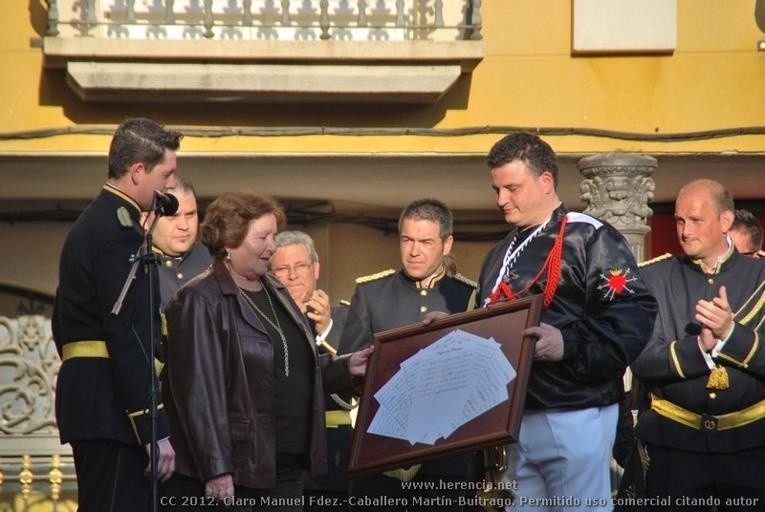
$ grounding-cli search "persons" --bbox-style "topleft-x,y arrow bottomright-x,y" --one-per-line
420,132 -> 658,512
160,189 -> 374,511
52,116 -> 186,511
727,208 -> 765,260
270,227 -> 353,511
141,175 -> 216,311
633,176 -> 765,510
341,199 -> 479,511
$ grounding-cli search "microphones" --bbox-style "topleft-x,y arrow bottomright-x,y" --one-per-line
151,190 -> 179,216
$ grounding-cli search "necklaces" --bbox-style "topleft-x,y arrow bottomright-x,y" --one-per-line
237,280 -> 290,380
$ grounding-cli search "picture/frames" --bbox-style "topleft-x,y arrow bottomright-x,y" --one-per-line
346,291 -> 544,473
